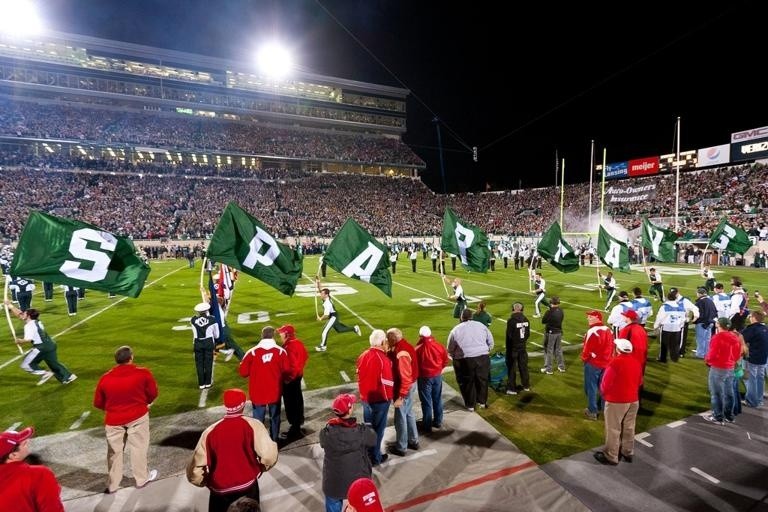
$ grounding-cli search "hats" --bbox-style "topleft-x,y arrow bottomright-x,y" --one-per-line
586,311 -> 602,321
619,291 -> 628,298
223,388 -> 247,413
713,284 -> 723,289
697,286 -> 708,291
419,326 -> 432,336
0,426 -> 34,459
276,324 -> 295,335
194,302 -> 211,313
551,296 -> 560,304
718,317 -> 732,330
670,288 -> 677,293
613,338 -> 633,353
332,393 -> 357,415
730,282 -> 742,288
621,309 -> 637,320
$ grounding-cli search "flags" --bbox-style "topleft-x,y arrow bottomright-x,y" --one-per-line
594,224 -> 632,276
321,216 -> 394,300
5,209 -> 151,299
536,220 -> 580,275
204,200 -> 304,299
708,218 -> 753,256
440,204 -> 489,276
641,213 -> 679,264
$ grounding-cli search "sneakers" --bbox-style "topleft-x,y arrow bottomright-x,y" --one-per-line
522,386 -> 530,391
388,445 -> 406,456
225,348 -> 236,362
620,453 -> 633,462
463,404 -> 475,411
406,442 -> 420,451
353,324 -> 362,337
540,368 -> 554,374
62,373 -> 78,384
199,385 -> 206,389
703,414 -> 726,425
532,314 -> 541,319
36,370 -> 55,386
373,453 -> 388,467
206,379 -> 213,388
478,402 -> 486,407
506,389 -> 517,395
137,469 -> 158,487
557,365 -> 565,372
315,345 -> 328,352
583,407 -> 597,421
594,451 -> 617,466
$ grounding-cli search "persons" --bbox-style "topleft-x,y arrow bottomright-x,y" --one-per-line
58,285 -> 84,315
530,273 -> 551,319
33,180 -> 227,238
703,317 -> 744,426
727,162 -> 768,241
227,180 -> 444,235
41,281 -> 54,302
1,66 -> 426,181
356,329 -> 388,467
506,302 -> 530,395
319,394 -> 377,512
631,287 -> 654,329
6,276 -> 22,304
444,160 -> 641,235
387,328 -> 420,457
1,180 -> 32,242
238,326 -> 291,445
227,496 -> 261,512
314,288 -> 361,352
446,309 -> 494,412
607,291 -> 644,339
641,168 -> 708,241
276,324 -> 308,439
653,292 -> 686,363
598,272 -> 622,313
443,276 -> 468,318
618,309 -> 648,415
685,245 -> 695,265
4,300 -> 77,386
580,310 -> 615,421
541,296 -> 566,375
415,326 -> 449,435
207,297 -> 246,365
134,240 -> 203,269
597,272 -> 619,312
713,283 -> 732,319
643,267 -> 665,304
0,426 -> 65,512
193,303 -> 220,389
186,389 -> 278,512
730,281 -> 749,332
299,235 -> 329,276
563,236 -> 592,266
490,237 -> 544,272
691,289 -> 718,359
709,166 -> 726,229
77,287 -> 86,300
720,249 -> 768,270
387,237 -> 441,278
669,287 -> 700,358
739,310 -> 767,409
472,300 -> 493,328
700,264 -> 716,297
93,346 -> 159,493
594,339 -> 643,466
7,278 -> 36,312
